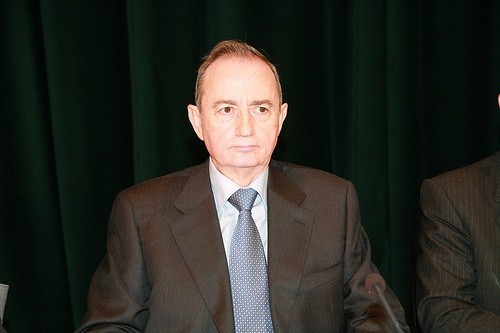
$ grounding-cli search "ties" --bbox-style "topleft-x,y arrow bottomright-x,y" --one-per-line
227,188 -> 274,333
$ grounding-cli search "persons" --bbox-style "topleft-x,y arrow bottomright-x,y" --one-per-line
77,40 -> 411,333
413,90 -> 500,333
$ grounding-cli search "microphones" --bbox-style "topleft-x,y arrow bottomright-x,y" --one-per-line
365,274 -> 405,333
